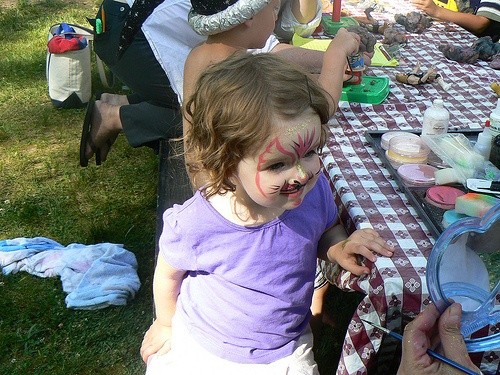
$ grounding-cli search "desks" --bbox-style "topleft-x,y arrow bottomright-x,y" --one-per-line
275,0 -> 500,375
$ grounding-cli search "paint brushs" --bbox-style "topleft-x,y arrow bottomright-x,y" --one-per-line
347,56 -> 355,76
360,319 -> 481,375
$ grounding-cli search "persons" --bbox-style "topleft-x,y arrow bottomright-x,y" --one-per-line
408,0 -> 500,44
396,298 -> 484,375
139,48 -> 394,375
80,0 -> 322,164
182,0 -> 360,199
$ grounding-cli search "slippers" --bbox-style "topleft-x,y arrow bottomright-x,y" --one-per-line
96,90 -> 119,165
80,98 -> 99,167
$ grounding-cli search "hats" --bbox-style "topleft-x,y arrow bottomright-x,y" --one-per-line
188,0 -> 272,36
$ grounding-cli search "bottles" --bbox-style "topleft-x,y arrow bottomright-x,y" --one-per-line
489,98 -> 500,140
475,121 -> 492,158
422,99 -> 449,136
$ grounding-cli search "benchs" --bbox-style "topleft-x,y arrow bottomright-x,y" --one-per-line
156,130 -> 193,267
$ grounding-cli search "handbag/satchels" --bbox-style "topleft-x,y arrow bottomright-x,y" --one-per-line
46,22 -> 115,108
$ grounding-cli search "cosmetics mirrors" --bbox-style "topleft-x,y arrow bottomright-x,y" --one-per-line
426,204 -> 500,353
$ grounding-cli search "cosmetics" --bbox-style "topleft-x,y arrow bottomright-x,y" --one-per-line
380,132 -> 500,229
